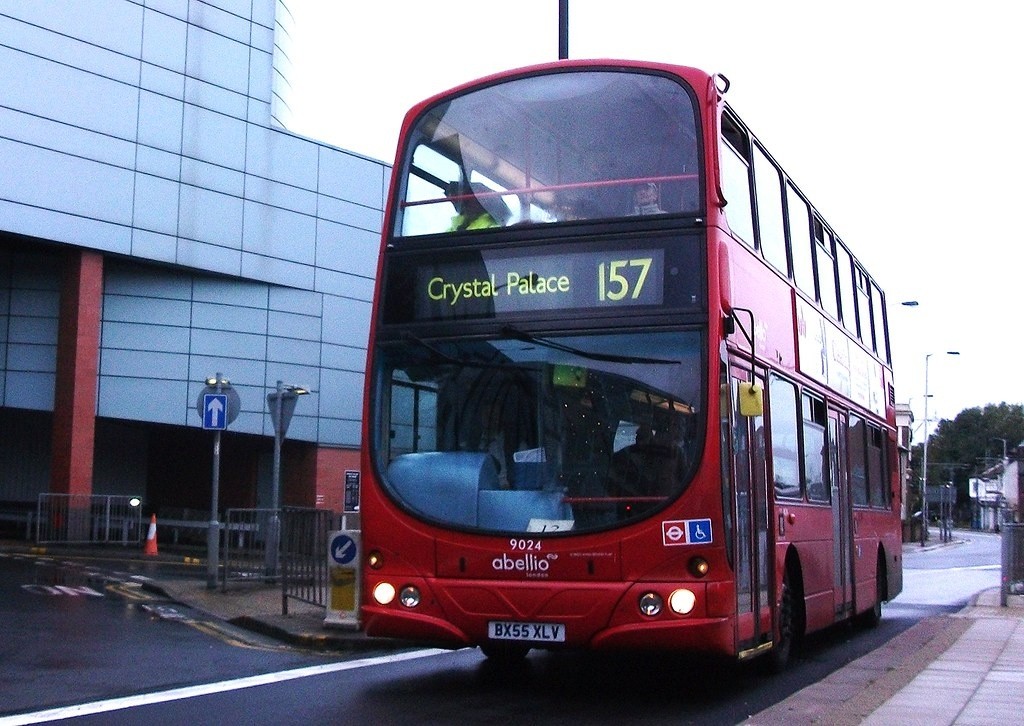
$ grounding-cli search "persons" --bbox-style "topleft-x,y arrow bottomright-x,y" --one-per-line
443,179 -> 505,232
625,181 -> 671,216
454,396 -> 536,490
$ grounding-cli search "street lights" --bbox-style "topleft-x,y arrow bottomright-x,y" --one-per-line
919,350 -> 961,548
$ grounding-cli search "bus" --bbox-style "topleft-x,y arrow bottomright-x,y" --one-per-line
359,56 -> 907,679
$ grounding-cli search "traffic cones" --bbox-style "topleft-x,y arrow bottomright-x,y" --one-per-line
144,514 -> 159,556
